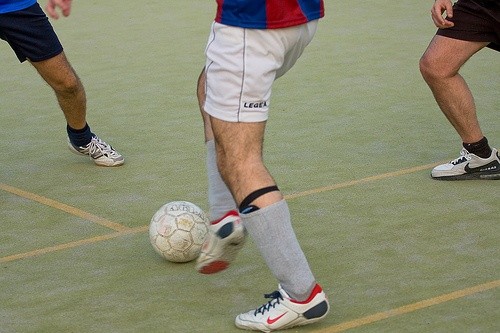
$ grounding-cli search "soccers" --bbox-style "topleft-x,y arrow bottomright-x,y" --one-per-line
149,200 -> 210,263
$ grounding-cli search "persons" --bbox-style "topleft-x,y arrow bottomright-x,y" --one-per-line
419,0 -> 500,181
0,0 -> 125,167
195,0 -> 330,333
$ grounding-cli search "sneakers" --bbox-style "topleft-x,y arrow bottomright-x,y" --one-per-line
235,283 -> 331,331
67,132 -> 125,167
431,147 -> 500,180
195,210 -> 248,275
